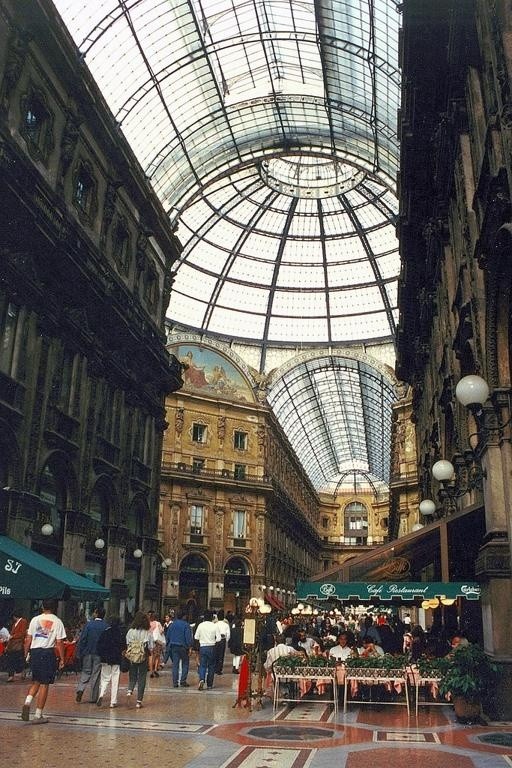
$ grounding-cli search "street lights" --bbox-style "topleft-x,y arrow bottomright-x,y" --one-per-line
313,608 -> 318,624
259,603 -> 272,633
298,603 -> 304,620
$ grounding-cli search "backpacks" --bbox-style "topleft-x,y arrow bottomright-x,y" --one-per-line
124,640 -> 145,664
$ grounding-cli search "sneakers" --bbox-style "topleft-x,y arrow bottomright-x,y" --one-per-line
150,671 -> 159,678
96,697 -> 103,706
174,681 -> 189,688
22,705 -> 29,721
136,702 -> 141,708
198,680 -> 204,691
126,692 -> 132,696
32,716 -> 48,724
76,692 -> 83,702
109,704 -> 117,708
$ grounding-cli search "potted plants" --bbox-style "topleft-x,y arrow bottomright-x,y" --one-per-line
262,638 -> 503,728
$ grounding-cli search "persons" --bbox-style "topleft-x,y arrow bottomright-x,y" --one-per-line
274,611 -> 412,696
0,624 -> 10,643
161,609 -> 244,691
96,615 -> 126,708
7,610 -> 28,682
126,610 -> 153,709
21,599 -> 67,724
146,613 -> 164,677
148,611 -> 154,617
75,608 -> 111,703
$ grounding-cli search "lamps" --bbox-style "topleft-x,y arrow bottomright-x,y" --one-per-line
419,499 -> 439,525
432,458 -> 488,498
455,374 -> 512,433
81,538 -> 104,552
153,557 -> 172,570
120,546 -> 143,562
25,521 -> 54,541
